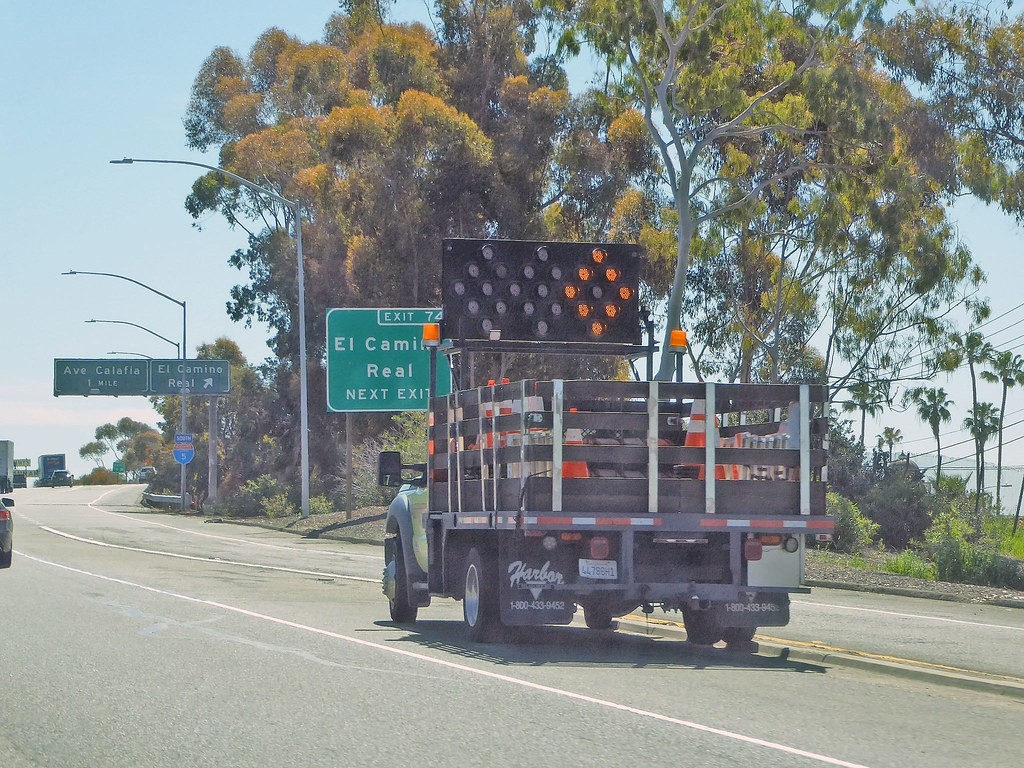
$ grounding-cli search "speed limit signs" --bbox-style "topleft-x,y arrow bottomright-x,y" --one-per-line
173,441 -> 194,465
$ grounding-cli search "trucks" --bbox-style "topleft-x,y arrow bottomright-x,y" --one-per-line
376,237 -> 837,648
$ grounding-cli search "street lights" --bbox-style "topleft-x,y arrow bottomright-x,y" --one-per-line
109,156 -> 309,519
84,318 -> 180,360
60,269 -> 187,516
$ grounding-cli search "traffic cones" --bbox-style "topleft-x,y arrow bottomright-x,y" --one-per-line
561,407 -> 590,478
498,376 -> 521,450
683,398 -> 725,479
479,379 -> 503,450
525,381 -> 548,435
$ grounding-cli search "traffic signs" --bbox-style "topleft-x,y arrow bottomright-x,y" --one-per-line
325,306 -> 453,413
54,357 -> 231,396
175,434 -> 193,442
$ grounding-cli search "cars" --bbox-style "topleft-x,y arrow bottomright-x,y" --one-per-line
139,467 -> 157,484
50,470 -> 74,488
0,497 -> 15,568
